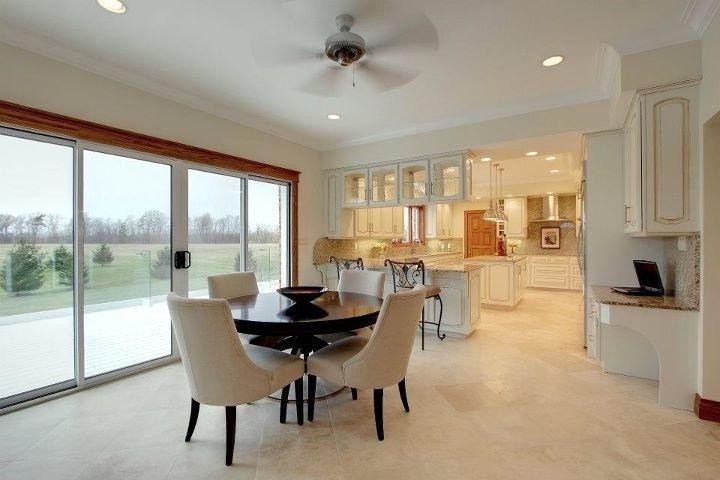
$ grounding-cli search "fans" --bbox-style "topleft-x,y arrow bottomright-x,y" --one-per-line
246,0 -> 440,100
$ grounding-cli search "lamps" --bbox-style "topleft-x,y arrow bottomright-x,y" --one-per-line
483,162 -> 498,220
499,168 -> 508,222
493,164 -> 504,221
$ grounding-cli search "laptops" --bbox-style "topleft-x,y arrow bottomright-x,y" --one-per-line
611,259 -> 665,296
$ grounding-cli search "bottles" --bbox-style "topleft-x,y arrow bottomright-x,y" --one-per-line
497,237 -> 505,256
503,234 -> 508,256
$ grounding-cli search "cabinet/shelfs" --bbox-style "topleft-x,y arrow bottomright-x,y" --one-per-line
326,175 -> 404,239
622,76 -> 700,233
535,257 -> 582,290
504,197 -> 527,237
463,255 -> 525,311
425,199 -> 452,240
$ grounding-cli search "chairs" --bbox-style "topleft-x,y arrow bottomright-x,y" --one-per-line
167,291 -> 305,466
306,282 -> 427,441
314,270 -> 385,344
329,256 -> 374,331
384,258 -> 446,350
207,271 -> 284,405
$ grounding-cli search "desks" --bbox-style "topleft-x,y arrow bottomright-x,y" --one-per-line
224,291 -> 384,423
589,285 -> 700,412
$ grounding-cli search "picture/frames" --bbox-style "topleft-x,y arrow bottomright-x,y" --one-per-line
541,227 -> 560,249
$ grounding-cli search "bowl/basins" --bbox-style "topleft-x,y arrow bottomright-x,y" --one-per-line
276,286 -> 329,303
